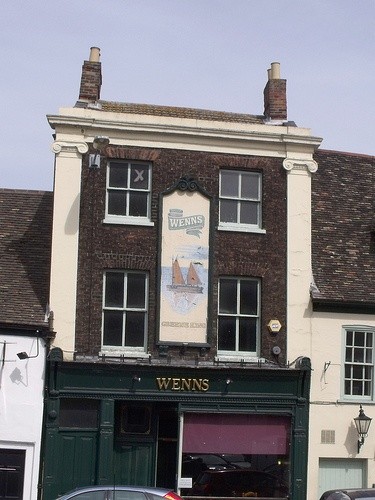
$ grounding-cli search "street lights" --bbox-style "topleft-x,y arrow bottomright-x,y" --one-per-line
354,404 -> 372,454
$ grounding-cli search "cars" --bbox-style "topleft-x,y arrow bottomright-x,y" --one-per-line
53,485 -> 185,500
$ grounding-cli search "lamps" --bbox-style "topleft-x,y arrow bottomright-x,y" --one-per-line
353,403 -> 373,454
87,135 -> 110,169
16,330 -> 41,359
